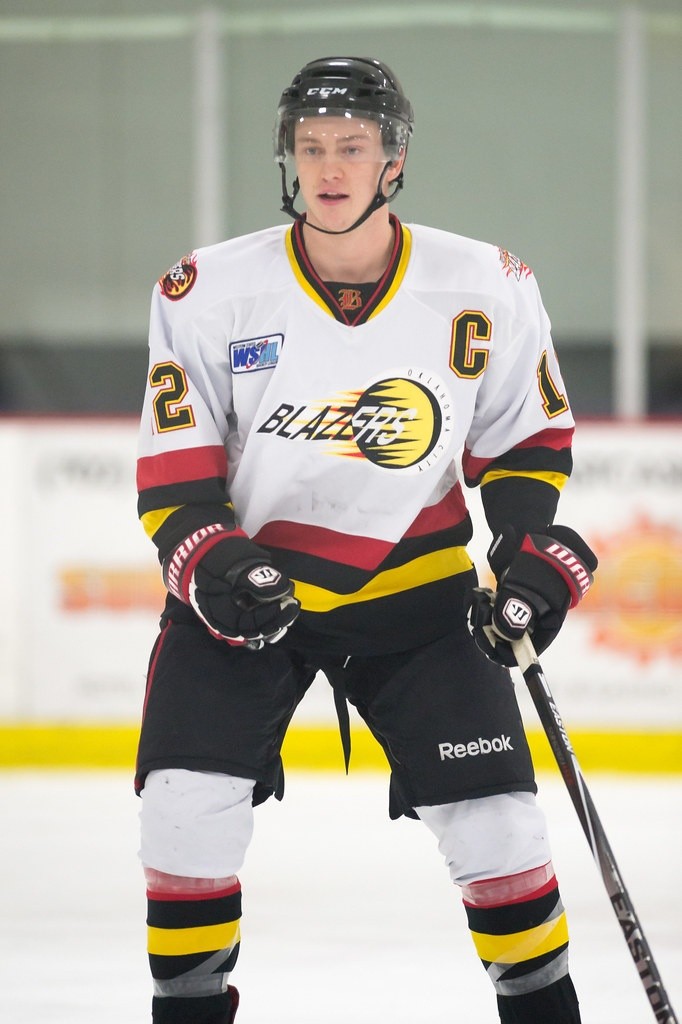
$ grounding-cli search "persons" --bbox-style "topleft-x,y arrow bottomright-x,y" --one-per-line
134,55 -> 600,1024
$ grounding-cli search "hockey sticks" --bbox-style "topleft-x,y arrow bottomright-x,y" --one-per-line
487,569 -> 678,1024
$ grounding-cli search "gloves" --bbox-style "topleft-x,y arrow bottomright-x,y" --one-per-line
161,523 -> 301,650
468,524 -> 597,667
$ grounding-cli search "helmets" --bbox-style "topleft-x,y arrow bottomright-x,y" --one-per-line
278,55 -> 414,140
